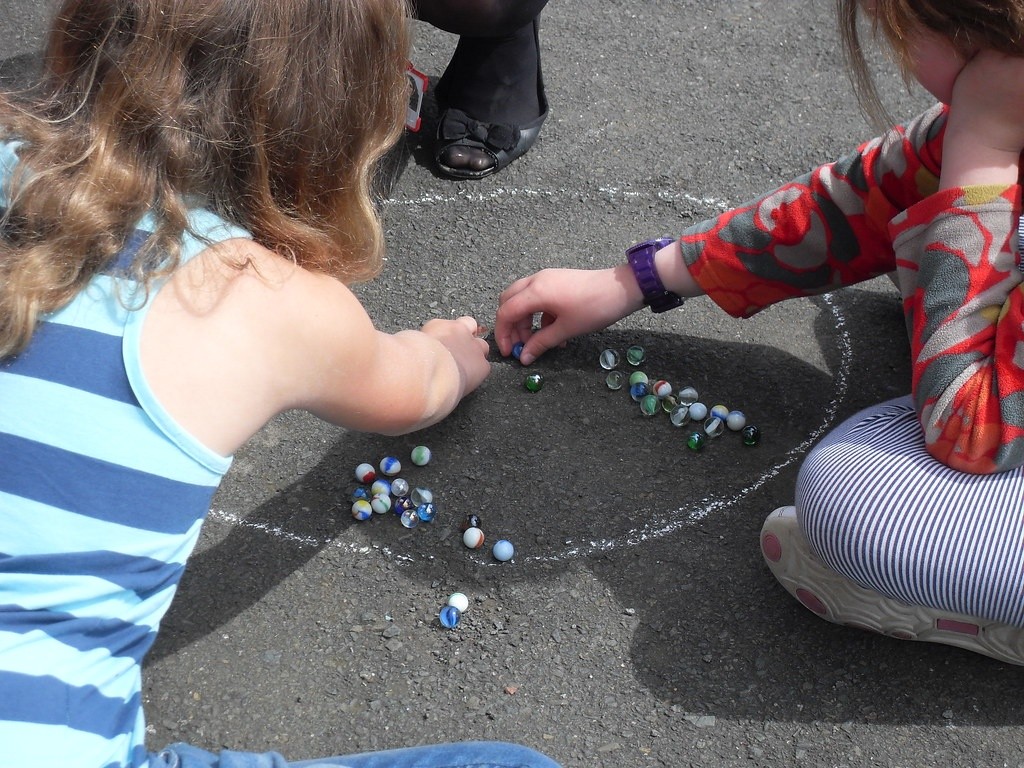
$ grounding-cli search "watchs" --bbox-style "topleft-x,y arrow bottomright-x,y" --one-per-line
626,236 -> 685,313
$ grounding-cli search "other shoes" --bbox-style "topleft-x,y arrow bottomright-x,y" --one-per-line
759,507 -> 1024,667
434,10 -> 549,177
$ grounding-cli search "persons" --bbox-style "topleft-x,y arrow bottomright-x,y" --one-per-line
0,0 -> 558,766
405,0 -> 549,180
494,0 -> 1024,664
